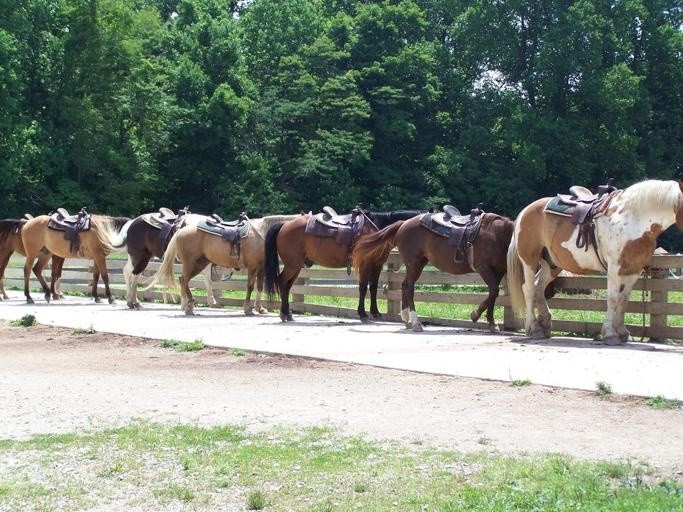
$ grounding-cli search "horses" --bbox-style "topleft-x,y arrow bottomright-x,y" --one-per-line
87,209 -> 242,311
351,209 -> 557,334
504,177 -> 683,348
263,207 -> 439,326
140,208 -> 313,317
0,216 -> 67,303
19,212 -> 134,306
